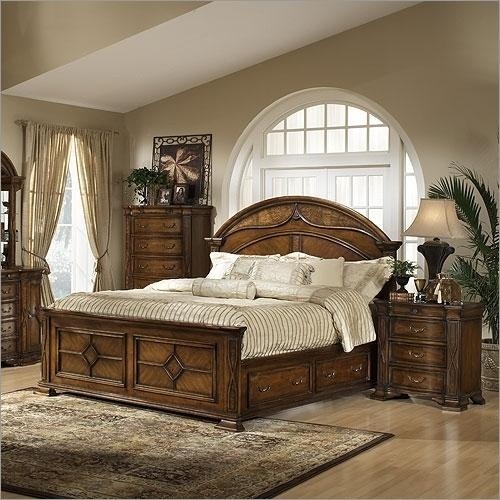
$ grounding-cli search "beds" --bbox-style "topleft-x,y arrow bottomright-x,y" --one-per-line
33,195 -> 397,431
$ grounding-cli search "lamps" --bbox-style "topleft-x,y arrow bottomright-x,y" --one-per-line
400,197 -> 469,302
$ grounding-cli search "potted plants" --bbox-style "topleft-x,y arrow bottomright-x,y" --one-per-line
385,255 -> 421,294
123,168 -> 170,206
425,160 -> 499,395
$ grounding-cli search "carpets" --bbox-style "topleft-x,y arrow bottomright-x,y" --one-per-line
0,383 -> 396,499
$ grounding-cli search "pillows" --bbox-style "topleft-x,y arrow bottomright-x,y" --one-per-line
338,256 -> 395,304
288,256 -> 345,288
207,249 -> 281,280
222,255 -> 315,287
192,278 -> 257,301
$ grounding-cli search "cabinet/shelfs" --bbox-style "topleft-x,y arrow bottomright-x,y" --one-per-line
120,206 -> 213,288
1,266 -> 50,368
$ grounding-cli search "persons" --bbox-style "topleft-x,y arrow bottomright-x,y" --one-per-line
164,191 -> 170,202
138,190 -> 145,205
176,188 -> 184,201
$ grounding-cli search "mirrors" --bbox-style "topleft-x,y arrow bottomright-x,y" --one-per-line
1,150 -> 26,271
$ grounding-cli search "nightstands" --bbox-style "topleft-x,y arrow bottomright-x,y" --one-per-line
368,299 -> 487,411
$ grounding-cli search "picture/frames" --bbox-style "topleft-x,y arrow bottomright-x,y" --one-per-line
151,133 -> 214,207
171,182 -> 190,204
